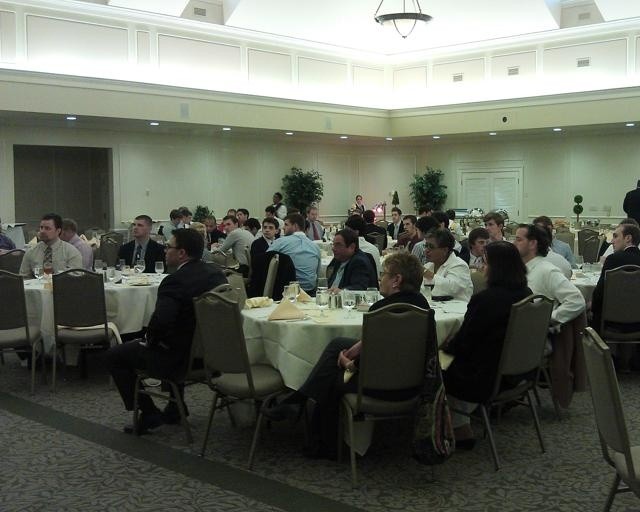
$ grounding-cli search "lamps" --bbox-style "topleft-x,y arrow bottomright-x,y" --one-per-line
374,1 -> 432,37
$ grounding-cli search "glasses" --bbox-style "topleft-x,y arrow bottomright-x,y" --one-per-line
165,243 -> 177,251
379,272 -> 394,277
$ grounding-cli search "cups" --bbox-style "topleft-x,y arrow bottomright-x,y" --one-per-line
565,261 -> 610,282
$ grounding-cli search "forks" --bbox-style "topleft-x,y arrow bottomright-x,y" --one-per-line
247,278 -> 467,326
21,261 -> 166,289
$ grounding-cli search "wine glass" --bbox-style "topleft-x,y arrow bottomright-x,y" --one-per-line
136,259 -> 145,277
576,255 -> 584,274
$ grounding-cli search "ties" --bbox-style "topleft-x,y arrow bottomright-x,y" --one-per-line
43,248 -> 52,264
135,245 -> 142,265
312,223 -> 320,240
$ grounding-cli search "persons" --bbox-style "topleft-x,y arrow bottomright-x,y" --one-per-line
58,217 -> 93,271
438,240 -> 533,451
106,228 -> 229,435
259,253 -> 434,461
115,192 -> 576,301
509,223 -> 584,331
0,216 -> 21,256
591,181 -> 638,353
16,212 -> 82,370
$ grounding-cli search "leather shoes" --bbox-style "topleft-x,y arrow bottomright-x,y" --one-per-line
124,409 -> 166,433
259,399 -> 303,425
164,400 -> 190,417
